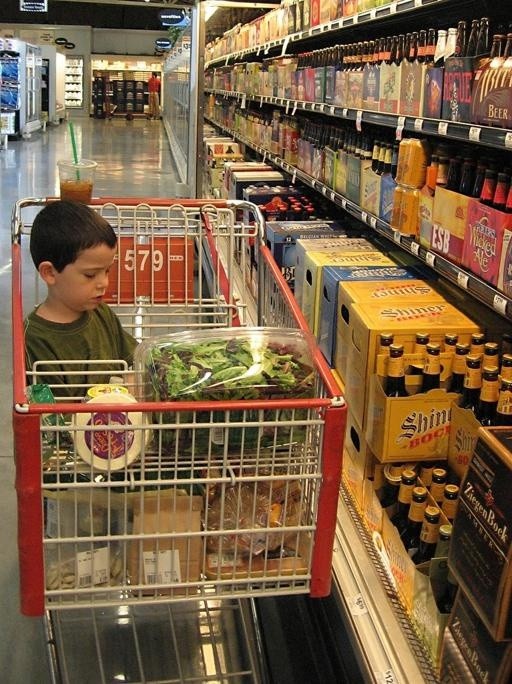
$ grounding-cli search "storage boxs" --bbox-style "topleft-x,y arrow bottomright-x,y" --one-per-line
117,495 -> 202,595
132,326 -> 318,458
202,124 -> 483,482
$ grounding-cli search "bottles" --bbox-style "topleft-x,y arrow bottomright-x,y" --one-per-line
380,330 -> 512,621
198,15 -> 512,225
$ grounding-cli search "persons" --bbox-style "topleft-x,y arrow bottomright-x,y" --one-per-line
147,72 -> 161,120
22,199 -> 205,496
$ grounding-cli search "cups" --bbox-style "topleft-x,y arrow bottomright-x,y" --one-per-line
57,158 -> 98,204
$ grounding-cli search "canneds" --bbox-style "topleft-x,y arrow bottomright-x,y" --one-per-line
390,137 -> 429,237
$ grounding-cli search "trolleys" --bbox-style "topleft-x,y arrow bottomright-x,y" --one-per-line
103,74 -> 150,122
10,196 -> 348,683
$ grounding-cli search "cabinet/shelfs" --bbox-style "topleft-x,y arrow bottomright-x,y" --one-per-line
65,57 -> 146,116
203,0 -> 512,684
